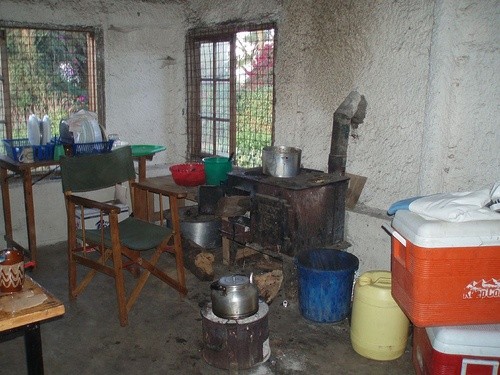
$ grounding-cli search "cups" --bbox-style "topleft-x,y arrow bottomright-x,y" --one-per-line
0,249 -> 25,292
107,134 -> 121,151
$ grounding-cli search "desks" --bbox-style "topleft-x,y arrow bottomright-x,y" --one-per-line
142,175 -> 200,223
0,273 -> 65,375
0,153 -> 153,271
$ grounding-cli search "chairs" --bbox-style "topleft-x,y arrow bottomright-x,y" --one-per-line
59,145 -> 189,327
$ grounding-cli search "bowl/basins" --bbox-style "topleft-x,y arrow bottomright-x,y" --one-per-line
169,164 -> 205,185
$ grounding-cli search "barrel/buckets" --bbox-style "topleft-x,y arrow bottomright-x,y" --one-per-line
163,206 -> 222,251
350,271 -> 409,361
202,156 -> 234,185
262,146 -> 302,178
295,248 -> 359,323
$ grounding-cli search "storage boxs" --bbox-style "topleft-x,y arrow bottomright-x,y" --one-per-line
55,137 -> 116,155
1,138 -> 56,163
412,325 -> 500,375
382,210 -> 499,327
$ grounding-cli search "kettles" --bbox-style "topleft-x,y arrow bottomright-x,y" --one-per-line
210,272 -> 258,319
